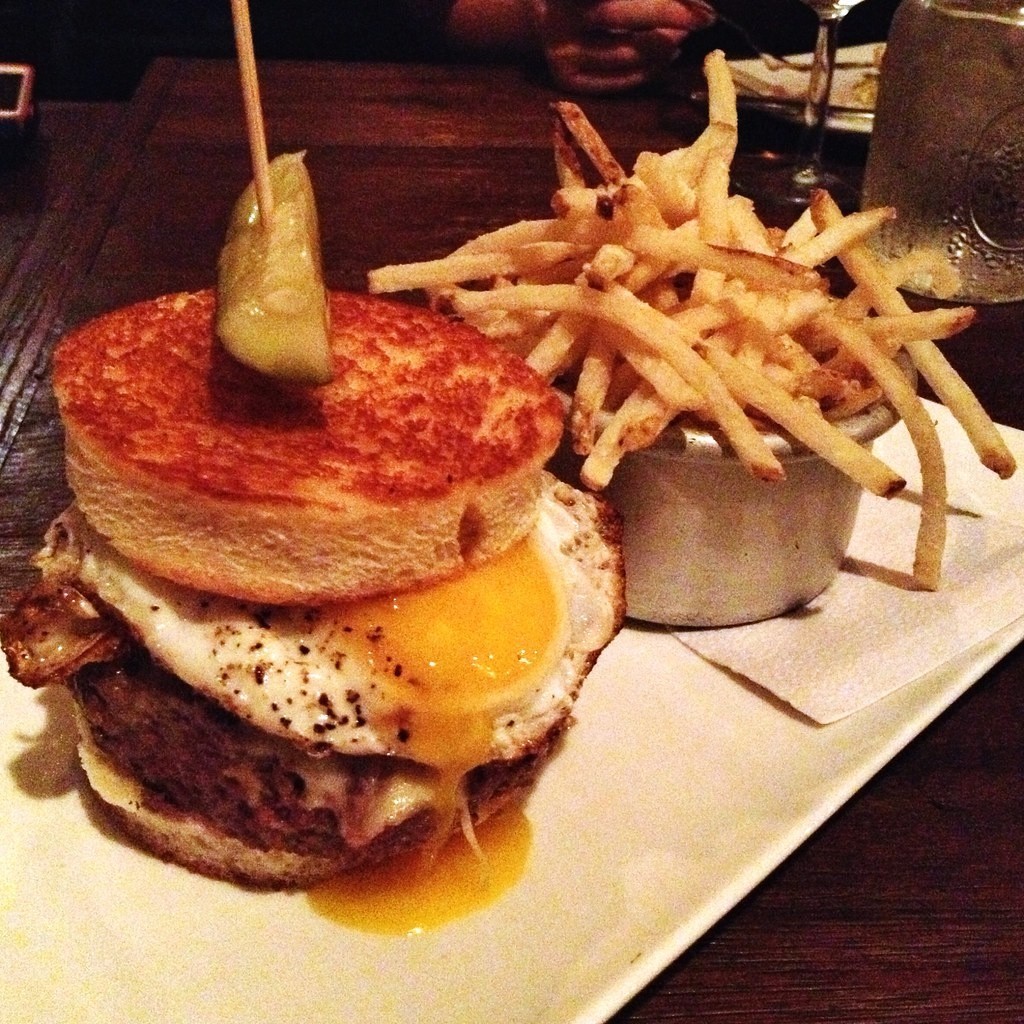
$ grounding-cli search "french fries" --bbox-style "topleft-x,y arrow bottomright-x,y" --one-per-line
369,50 -> 1017,595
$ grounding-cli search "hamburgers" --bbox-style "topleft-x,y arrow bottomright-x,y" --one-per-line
0,151 -> 633,893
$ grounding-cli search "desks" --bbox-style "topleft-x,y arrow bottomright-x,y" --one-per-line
0,56 -> 1024,1024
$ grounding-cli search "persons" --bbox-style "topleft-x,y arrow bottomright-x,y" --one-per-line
444,0 -> 717,92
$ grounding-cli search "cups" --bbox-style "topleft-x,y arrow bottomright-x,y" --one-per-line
855,0 -> 1024,307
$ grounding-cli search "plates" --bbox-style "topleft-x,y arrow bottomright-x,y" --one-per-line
723,40 -> 886,135
0,393 -> 1023,1024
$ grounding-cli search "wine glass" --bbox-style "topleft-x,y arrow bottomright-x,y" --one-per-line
728,1 -> 861,207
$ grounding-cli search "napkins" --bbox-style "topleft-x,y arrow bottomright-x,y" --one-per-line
665,477 -> 1024,726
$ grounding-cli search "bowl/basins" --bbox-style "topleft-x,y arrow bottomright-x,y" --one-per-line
544,308 -> 919,627
